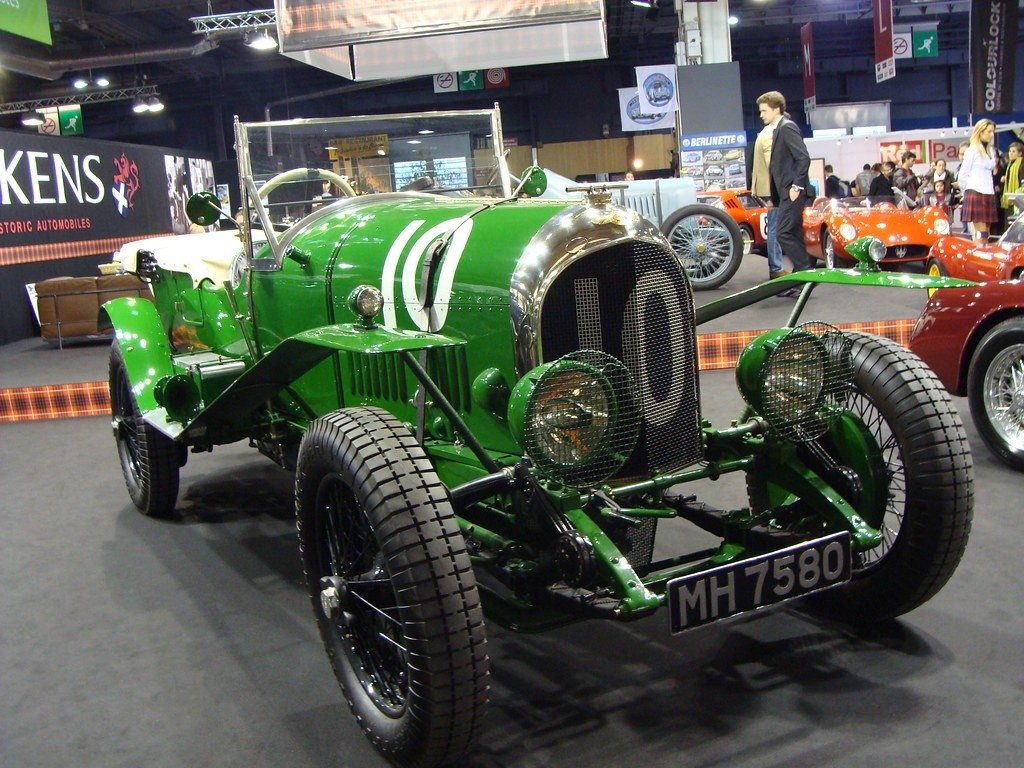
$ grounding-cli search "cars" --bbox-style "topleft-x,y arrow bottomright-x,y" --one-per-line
96,101 -> 988,768
696,187 -> 775,258
398,150 -> 747,293
911,212 -> 1023,473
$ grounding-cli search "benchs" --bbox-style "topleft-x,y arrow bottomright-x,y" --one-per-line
115,228 -> 283,351
34,272 -> 155,349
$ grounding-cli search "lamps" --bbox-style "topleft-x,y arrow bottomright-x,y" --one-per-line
191,30 -> 220,57
242,27 -> 278,50
148,96 -> 164,112
132,96 -> 149,114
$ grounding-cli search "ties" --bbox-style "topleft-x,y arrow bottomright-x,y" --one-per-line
772,129 -> 776,138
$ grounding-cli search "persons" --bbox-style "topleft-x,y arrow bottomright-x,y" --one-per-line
751,91 -> 813,297
824,141 -> 1024,242
958,119 -> 996,243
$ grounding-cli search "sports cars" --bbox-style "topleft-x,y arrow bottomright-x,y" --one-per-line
800,194 -> 951,268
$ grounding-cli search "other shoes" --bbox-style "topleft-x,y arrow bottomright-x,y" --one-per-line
777,288 -> 801,297
769,269 -> 790,280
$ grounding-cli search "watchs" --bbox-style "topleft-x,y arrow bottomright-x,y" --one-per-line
792,186 -> 799,192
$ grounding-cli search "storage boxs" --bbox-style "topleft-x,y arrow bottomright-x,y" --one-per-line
503,137 -> 518,146
477,138 -> 486,150
